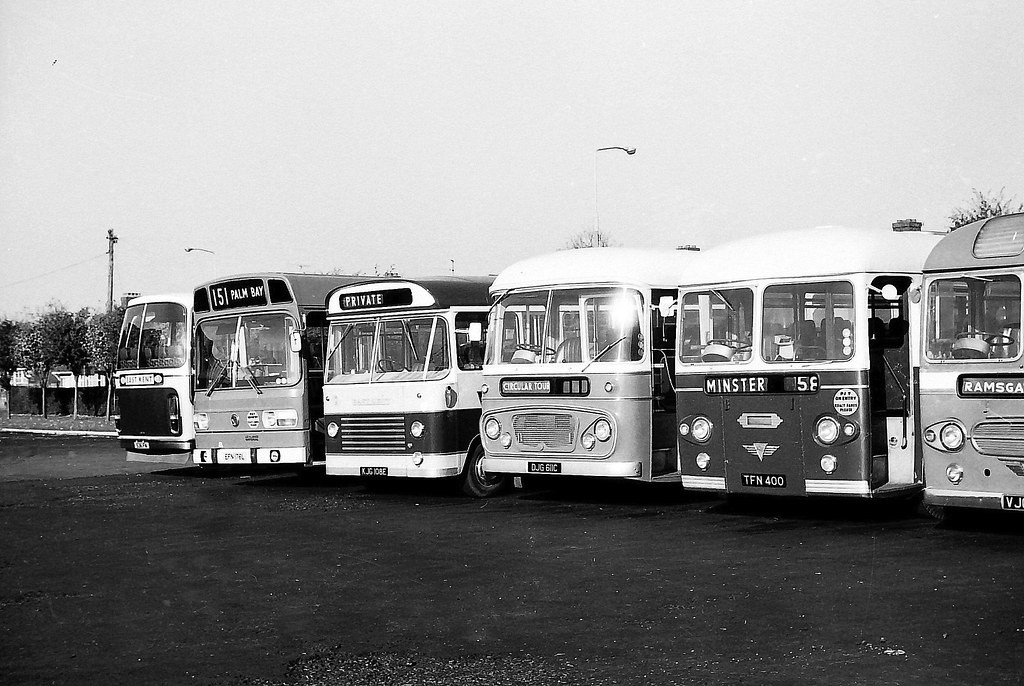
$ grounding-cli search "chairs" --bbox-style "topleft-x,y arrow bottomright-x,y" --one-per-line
765,318 -> 908,355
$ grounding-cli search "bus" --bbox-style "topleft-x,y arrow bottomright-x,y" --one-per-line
669,220 -> 954,515
912,209 -> 1024,526
112,289 -> 197,471
318,276 -> 499,500
180,273 -> 389,479
475,244 -> 705,498
